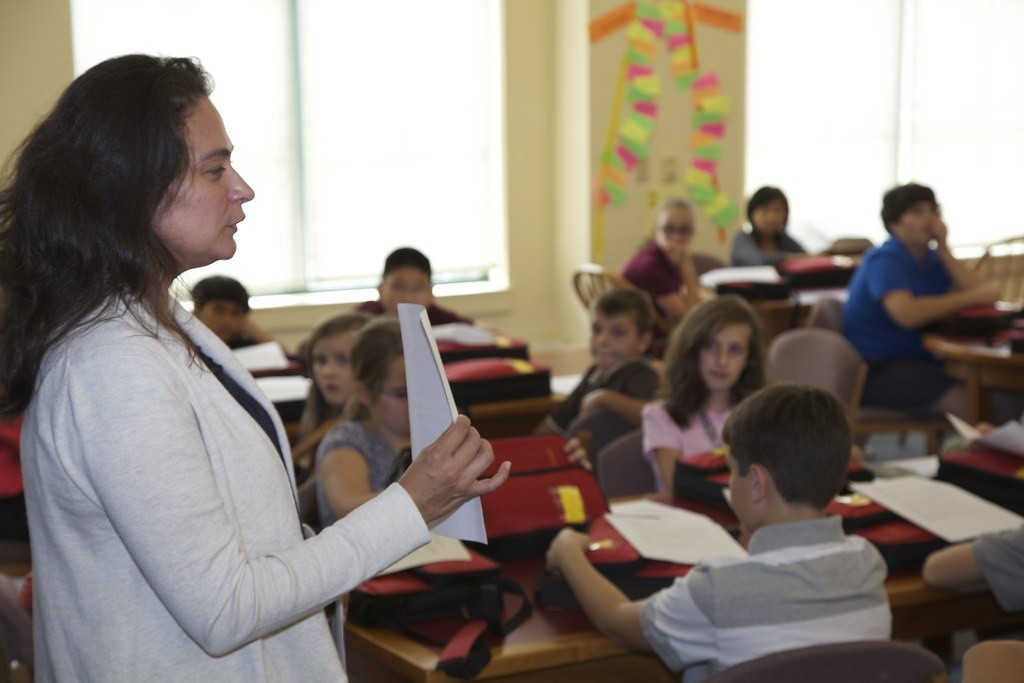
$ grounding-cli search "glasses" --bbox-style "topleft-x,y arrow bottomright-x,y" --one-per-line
659,224 -> 693,237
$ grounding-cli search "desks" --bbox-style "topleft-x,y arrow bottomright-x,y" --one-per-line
282,392 -> 569,449
923,329 -> 1023,428
324,475 -> 1024,682
252,357 -> 553,422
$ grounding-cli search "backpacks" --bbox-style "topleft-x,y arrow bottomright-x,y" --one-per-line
0,406 -> 29,530
783,253 -> 858,289
463,434 -> 610,560
818,460 -> 950,579
344,545 -> 534,680
935,443 -> 1024,517
431,321 -> 529,360
586,509 -> 696,595
923,301 -> 1024,353
442,356 -> 552,406
669,444 -> 736,517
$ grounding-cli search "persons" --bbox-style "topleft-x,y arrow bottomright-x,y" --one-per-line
526,175 -> 1004,510
919,523 -> 1024,610
189,246 -> 474,532
543,381 -> 897,683
1,52 -> 512,683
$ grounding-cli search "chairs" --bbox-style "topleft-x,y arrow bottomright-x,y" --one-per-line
697,639 -> 950,683
572,263 -> 676,365
962,640 -> 1024,683
763,326 -> 871,414
